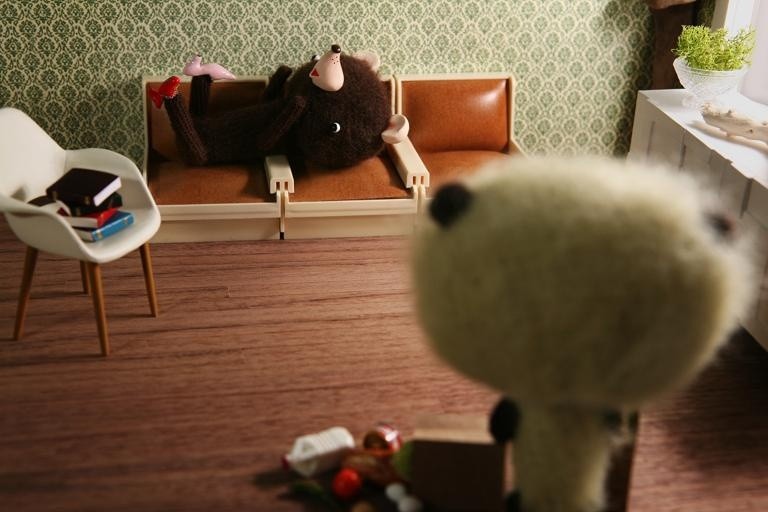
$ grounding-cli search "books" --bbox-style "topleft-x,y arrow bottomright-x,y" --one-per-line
46,166 -> 133,242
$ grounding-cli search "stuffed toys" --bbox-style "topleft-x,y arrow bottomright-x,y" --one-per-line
146,41 -> 410,176
408,147 -> 758,512
